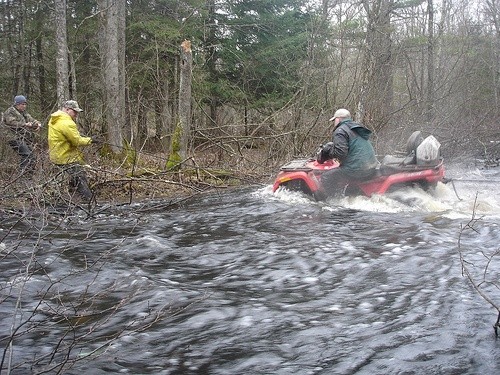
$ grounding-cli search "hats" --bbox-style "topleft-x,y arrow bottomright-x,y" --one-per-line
329,109 -> 350,121
13,95 -> 26,106
64,100 -> 84,112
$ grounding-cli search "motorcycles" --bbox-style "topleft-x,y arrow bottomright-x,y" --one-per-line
272,130 -> 452,204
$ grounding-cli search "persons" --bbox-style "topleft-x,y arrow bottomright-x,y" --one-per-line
47,99 -> 99,201
3,94 -> 42,184
320,109 -> 378,201
